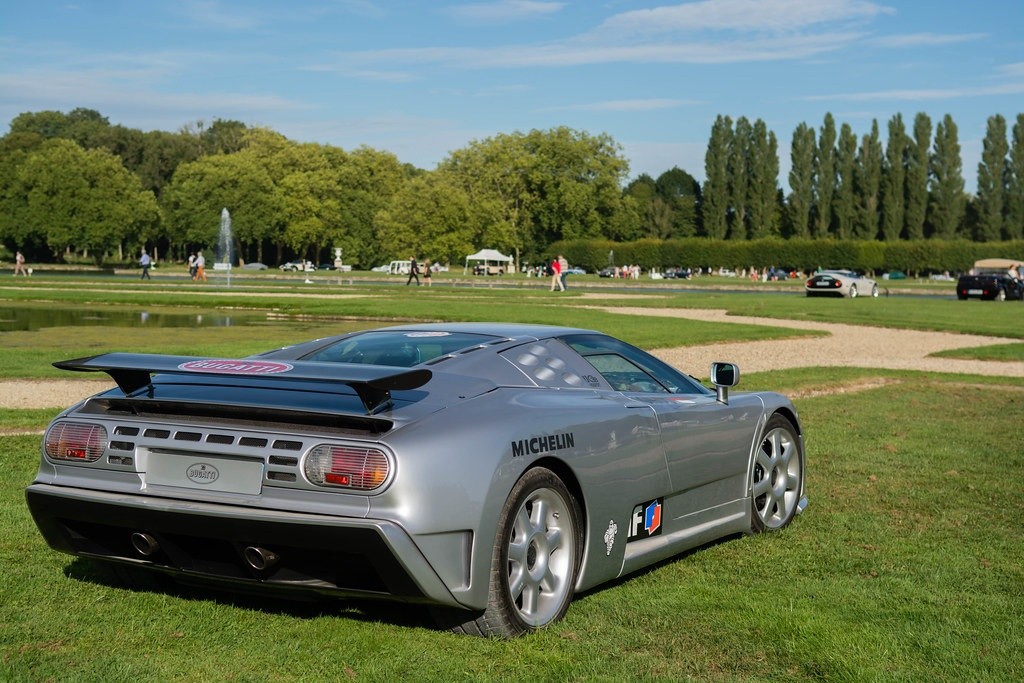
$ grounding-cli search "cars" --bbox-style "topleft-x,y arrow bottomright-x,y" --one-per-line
769,268 -> 789,280
663,266 -> 691,280
370,261 -> 442,274
597,266 -> 626,278
956,271 -> 1022,303
475,262 -> 503,277
565,266 -> 586,273
246,258 -> 339,271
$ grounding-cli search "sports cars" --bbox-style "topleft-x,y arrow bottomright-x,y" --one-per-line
24,323 -> 808,639
804,267 -> 880,299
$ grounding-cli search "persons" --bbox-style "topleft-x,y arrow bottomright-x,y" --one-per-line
9,248 -> 1023,298
